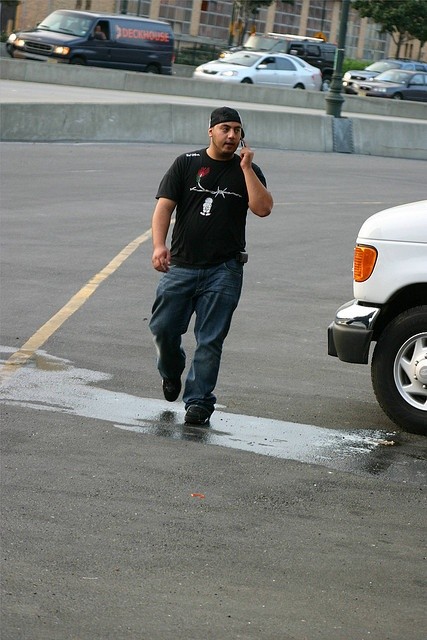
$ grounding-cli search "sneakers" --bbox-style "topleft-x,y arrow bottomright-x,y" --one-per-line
184,400 -> 214,426
162,373 -> 182,402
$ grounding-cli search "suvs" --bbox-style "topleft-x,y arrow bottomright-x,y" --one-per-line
327,198 -> 426,438
342,58 -> 427,94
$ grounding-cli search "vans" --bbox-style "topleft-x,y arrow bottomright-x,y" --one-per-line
5,8 -> 177,76
234,32 -> 338,77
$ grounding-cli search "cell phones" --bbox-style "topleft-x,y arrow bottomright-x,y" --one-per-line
240,127 -> 246,148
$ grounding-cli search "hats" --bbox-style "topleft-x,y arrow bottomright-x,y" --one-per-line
209,107 -> 245,140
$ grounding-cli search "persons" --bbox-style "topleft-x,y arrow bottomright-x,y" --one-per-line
148,106 -> 273,424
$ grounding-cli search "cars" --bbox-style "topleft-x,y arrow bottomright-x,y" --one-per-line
208,52 -> 323,91
352,69 -> 427,101
193,50 -> 315,91
